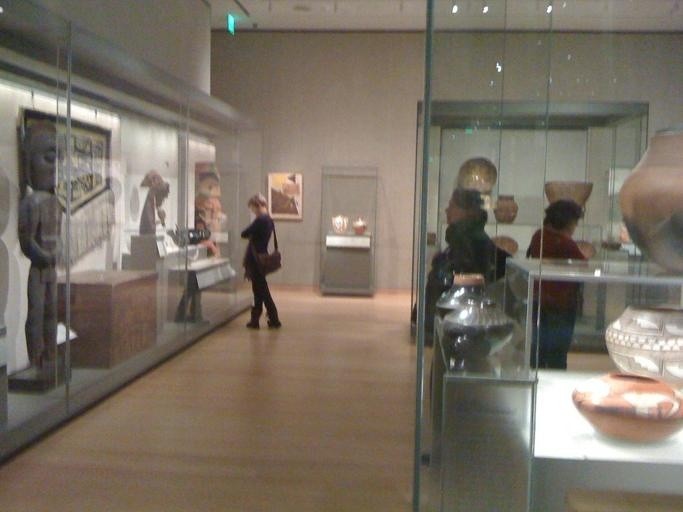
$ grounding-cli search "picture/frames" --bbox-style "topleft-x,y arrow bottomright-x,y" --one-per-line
267,170 -> 303,223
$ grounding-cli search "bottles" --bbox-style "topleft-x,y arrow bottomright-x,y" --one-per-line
603,303 -> 683,381
352,218 -> 366,235
618,126 -> 683,275
435,273 -> 516,366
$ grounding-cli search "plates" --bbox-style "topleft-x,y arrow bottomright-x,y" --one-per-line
457,158 -> 498,196
573,240 -> 597,259
489,236 -> 519,255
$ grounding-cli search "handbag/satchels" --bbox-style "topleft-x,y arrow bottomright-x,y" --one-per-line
251,249 -> 281,278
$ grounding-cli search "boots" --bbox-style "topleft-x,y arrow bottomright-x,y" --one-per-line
174,300 -> 190,322
265,309 -> 281,329
186,304 -> 210,324
245,306 -> 262,330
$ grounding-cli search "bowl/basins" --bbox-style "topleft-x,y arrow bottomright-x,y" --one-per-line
543,180 -> 593,208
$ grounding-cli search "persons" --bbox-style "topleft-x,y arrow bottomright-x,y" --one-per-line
174,191 -> 220,323
409,185 -> 510,472
239,190 -> 282,327
524,196 -> 592,374
17,118 -> 70,370
